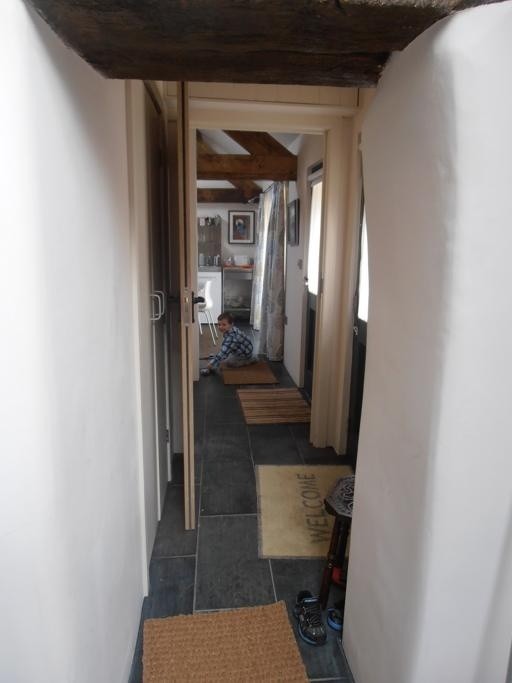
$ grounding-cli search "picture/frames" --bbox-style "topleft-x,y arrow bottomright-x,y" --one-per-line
228,209 -> 256,244
287,198 -> 301,247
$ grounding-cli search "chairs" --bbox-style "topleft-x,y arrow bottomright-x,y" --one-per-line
196,280 -> 219,346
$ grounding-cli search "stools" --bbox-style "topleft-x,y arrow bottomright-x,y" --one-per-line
314,470 -> 355,610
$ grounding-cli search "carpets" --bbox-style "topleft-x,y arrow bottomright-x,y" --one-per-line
141,600 -> 309,683
255,463 -> 355,562
222,364 -> 310,426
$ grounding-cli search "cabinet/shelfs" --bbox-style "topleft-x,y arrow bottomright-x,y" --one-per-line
198,272 -> 222,324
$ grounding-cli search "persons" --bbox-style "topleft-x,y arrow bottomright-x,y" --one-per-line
206,313 -> 257,369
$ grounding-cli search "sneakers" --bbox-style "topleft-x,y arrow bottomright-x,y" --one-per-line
292,590 -> 327,646
328,603 -> 344,632
